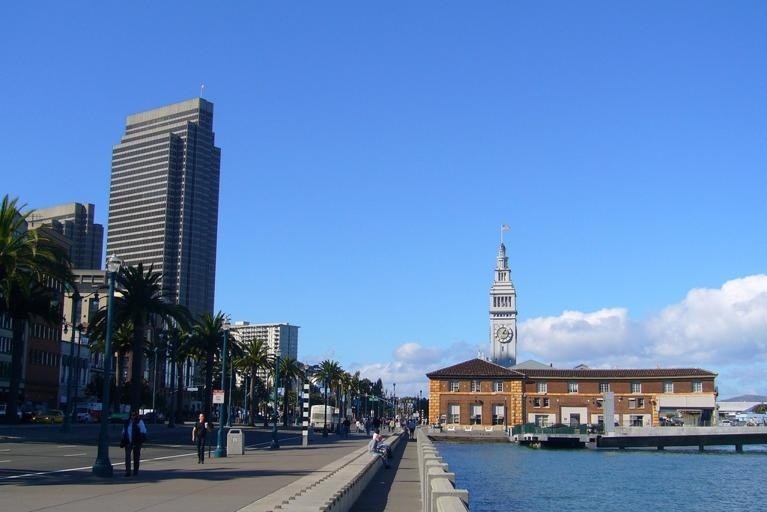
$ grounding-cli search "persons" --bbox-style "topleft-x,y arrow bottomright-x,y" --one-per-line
191,414 -> 210,464
122,410 -> 147,477
342,415 -> 427,468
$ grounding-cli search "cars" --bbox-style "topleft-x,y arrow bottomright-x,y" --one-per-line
142,412 -> 163,423
41,409 -> 64,424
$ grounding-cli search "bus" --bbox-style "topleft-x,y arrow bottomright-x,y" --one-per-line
310,405 -> 339,433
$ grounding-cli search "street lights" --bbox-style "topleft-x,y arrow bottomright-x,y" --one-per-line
64,289 -> 81,431
92,253 -> 122,477
270,348 -> 282,447
337,379 -> 369,433
213,317 -> 230,457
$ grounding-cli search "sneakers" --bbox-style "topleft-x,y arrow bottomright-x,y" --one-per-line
124,470 -> 138,477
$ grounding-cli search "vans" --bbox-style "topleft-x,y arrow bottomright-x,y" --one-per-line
73,407 -> 91,424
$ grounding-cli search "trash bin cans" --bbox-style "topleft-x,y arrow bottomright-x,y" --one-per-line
227,428 -> 244,455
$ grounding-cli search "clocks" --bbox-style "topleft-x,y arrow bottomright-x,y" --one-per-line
495,325 -> 513,343
